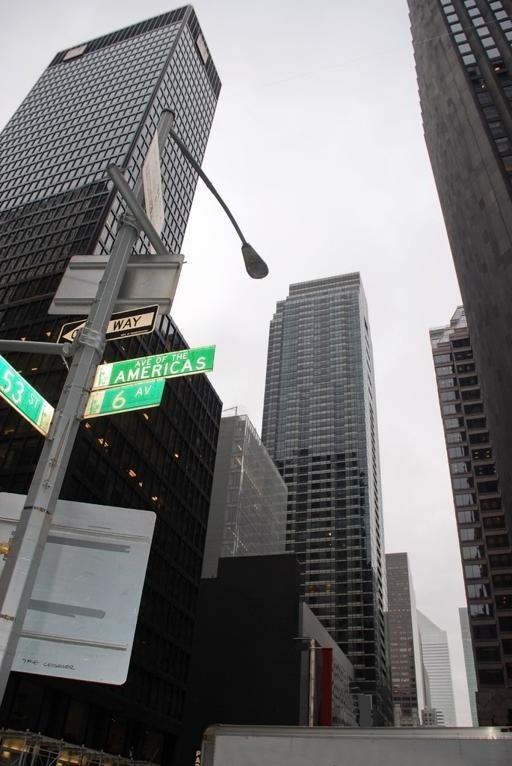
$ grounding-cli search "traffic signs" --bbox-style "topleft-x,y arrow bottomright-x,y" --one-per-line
92,344 -> 217,389
58,301 -> 159,346
140,128 -> 166,238
82,378 -> 165,425
0,351 -> 56,437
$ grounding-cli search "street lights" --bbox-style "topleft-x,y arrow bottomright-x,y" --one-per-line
0,107 -> 273,682
